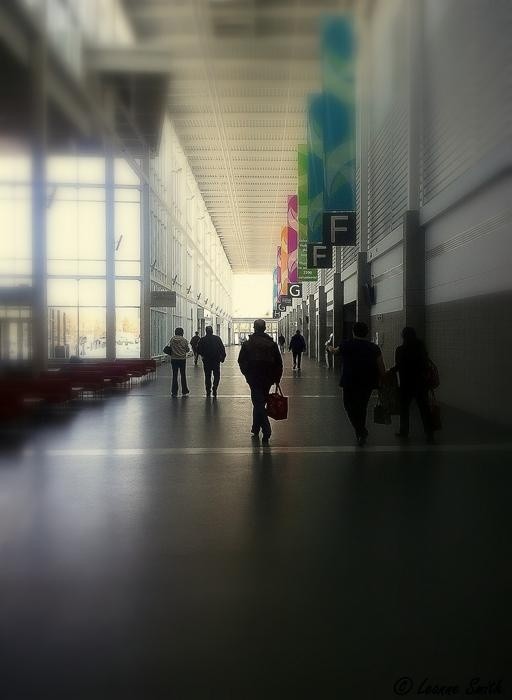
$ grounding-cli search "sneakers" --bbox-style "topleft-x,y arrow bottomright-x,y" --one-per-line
172,390 -> 189,396
251,429 -> 271,442
206,385 -> 217,396
395,432 -> 409,437
357,428 -> 368,446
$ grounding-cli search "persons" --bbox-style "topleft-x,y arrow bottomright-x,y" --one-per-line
198,325 -> 227,401
324,318 -> 388,443
167,326 -> 190,398
389,324 -> 439,443
190,331 -> 200,363
289,329 -> 307,370
238,318 -> 283,443
278,333 -> 286,354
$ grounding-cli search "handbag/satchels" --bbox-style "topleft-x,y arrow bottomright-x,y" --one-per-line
430,405 -> 441,430
373,373 -> 399,425
266,393 -> 288,420
163,346 -> 172,355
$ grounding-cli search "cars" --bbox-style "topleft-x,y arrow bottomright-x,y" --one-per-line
76,331 -> 141,356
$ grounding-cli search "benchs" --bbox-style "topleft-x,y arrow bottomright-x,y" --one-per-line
1,358 -> 157,424
